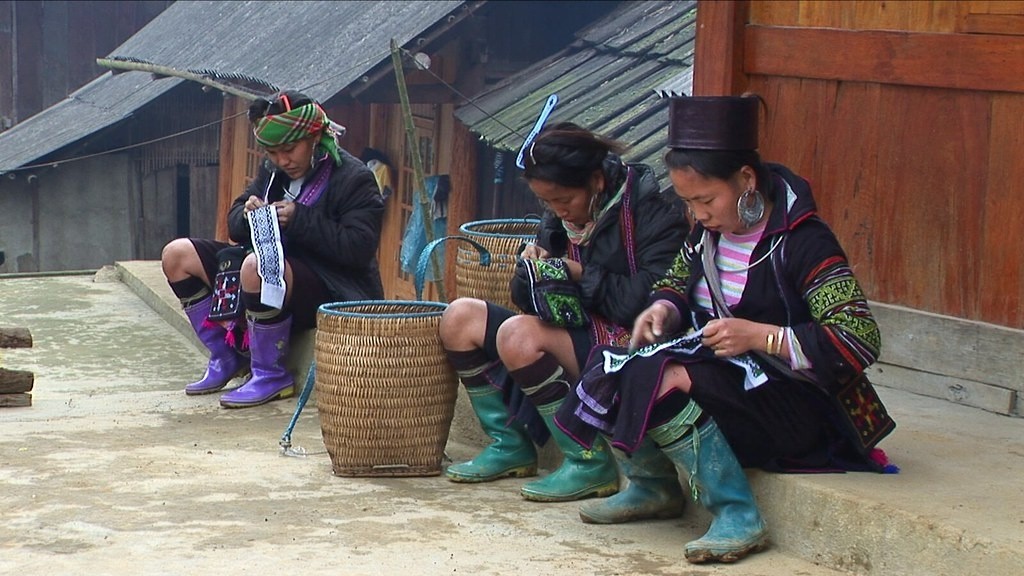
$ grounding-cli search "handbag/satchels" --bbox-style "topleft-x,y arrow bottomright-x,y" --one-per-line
822,371 -> 896,456
203,248 -> 256,349
587,311 -> 639,357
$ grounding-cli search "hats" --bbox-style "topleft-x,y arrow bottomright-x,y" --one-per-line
668,93 -> 760,150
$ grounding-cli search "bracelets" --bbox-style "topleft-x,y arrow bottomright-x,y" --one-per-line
767,334 -> 774,354
776,326 -> 785,354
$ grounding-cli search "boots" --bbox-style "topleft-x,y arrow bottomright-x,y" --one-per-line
220,308 -> 294,407
578,432 -> 697,523
520,396 -> 619,501
445,385 -> 539,481
660,415 -> 770,564
184,295 -> 253,395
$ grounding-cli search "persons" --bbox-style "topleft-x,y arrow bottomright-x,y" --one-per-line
438,122 -> 690,503
162,89 -> 383,407
553,95 -> 897,560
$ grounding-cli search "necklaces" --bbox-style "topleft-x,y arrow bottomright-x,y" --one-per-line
281,183 -> 295,200
715,234 -> 785,272
